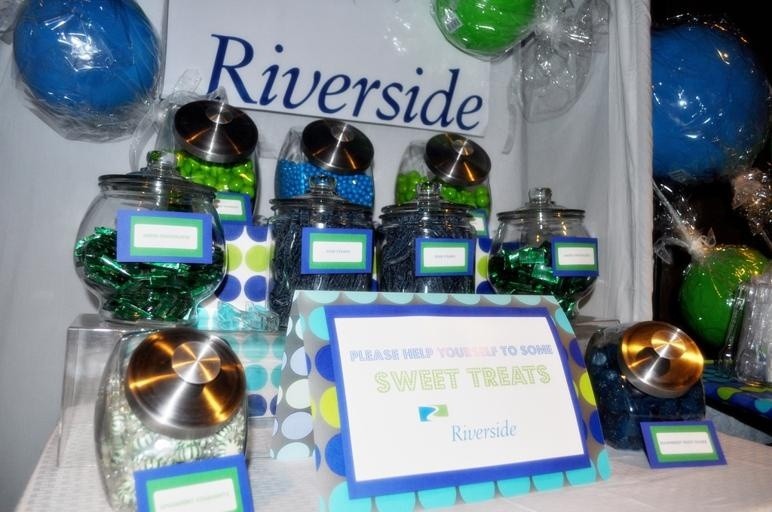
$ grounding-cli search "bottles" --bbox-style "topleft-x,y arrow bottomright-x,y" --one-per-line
72,151 -> 228,326
94,329 -> 247,509
376,182 -> 477,292
151,101 -> 259,222
275,119 -> 376,207
268,175 -> 373,329
396,134 -> 491,221
585,320 -> 705,451
486,186 -> 598,322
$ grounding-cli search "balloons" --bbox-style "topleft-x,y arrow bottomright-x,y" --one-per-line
14,1 -> 158,115
652,24 -> 760,177
680,246 -> 768,340
434,1 -> 538,55
